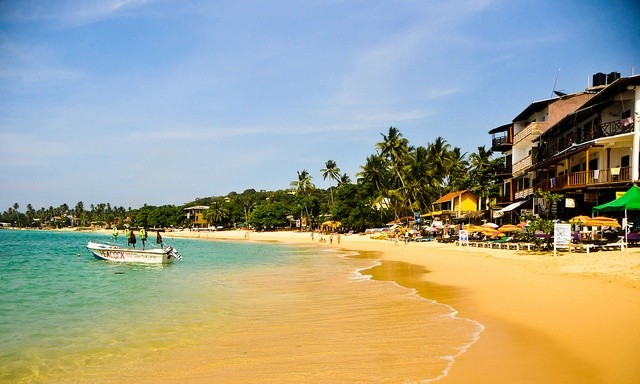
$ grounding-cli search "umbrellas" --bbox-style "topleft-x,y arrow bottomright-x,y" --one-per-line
237,223 -> 244,227
586,216 -> 621,238
498,224 -> 521,236
517,222 -> 526,227
569,214 -> 591,230
382,221 -> 506,239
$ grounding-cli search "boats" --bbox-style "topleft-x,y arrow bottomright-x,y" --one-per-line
86,239 -> 184,265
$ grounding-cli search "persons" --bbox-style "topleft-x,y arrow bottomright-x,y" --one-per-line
337,234 -> 341,243
128,230 -> 136,248
329,234 -> 333,243
404,229 -> 408,245
311,232 -> 314,240
157,231 -> 163,249
395,232 -> 399,245
